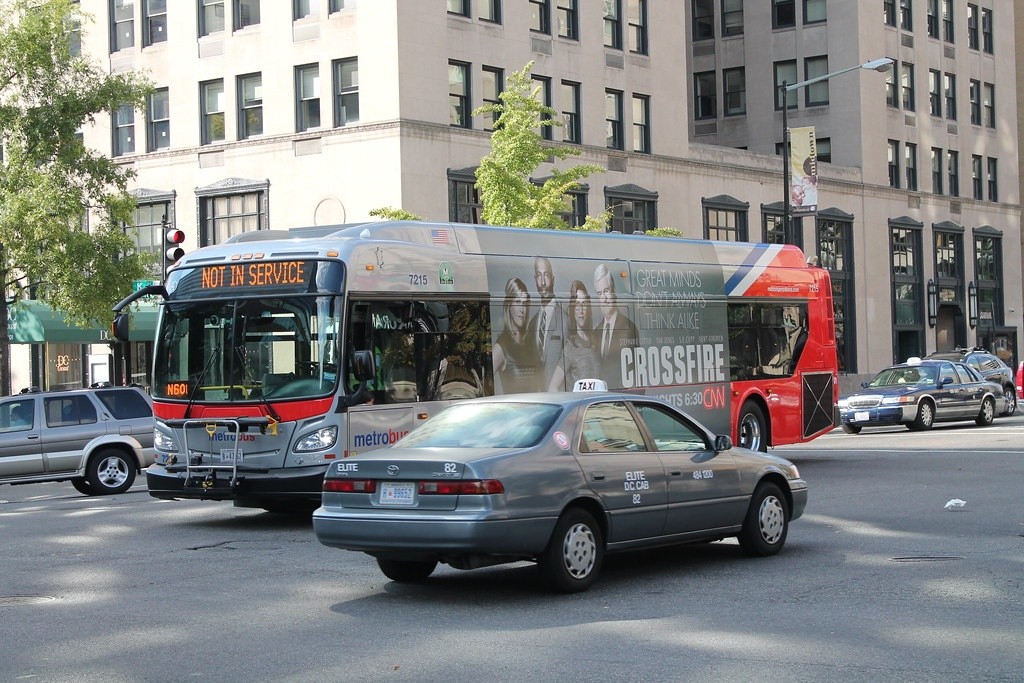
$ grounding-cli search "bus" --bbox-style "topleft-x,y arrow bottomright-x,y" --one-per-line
109,222 -> 838,517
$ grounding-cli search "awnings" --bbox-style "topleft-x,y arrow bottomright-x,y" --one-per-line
7,300 -> 159,345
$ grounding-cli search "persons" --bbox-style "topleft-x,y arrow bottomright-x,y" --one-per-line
768,305 -> 808,376
10,406 -> 29,427
792,174 -> 818,206
918,368 -> 934,385
348,323 -> 385,392
493,256 -> 646,394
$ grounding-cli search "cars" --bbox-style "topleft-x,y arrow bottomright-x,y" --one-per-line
312,378 -> 808,594
924,346 -> 1016,416
0,385 -> 156,496
837,355 -> 996,434
1016,361 -> 1024,413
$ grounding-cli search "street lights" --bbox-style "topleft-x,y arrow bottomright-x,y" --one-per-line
782,57 -> 894,245
163,226 -> 186,280
